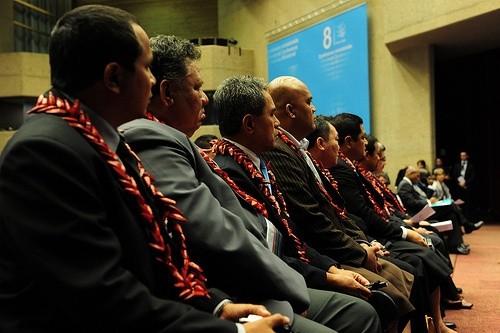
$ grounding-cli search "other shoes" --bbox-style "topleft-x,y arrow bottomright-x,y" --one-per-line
457,243 -> 470,254
465,221 -> 483,235
445,287 -> 474,333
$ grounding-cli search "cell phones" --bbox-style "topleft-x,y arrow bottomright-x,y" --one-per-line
381,241 -> 393,252
364,280 -> 388,291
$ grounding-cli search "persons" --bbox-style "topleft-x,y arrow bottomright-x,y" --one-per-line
0,4 -> 292,333
116,34 -> 382,333
191,76 -> 486,333
